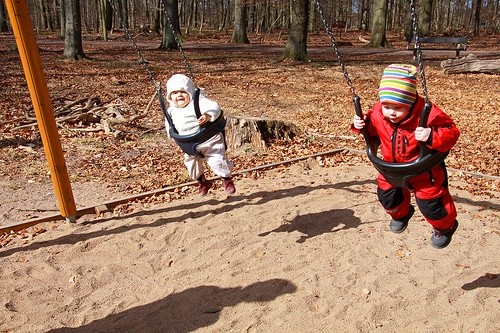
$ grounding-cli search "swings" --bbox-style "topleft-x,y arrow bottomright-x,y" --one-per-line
107,0 -> 229,159
314,1 -> 450,193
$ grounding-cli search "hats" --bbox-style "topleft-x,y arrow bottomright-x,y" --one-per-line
378,63 -> 419,108
165,73 -> 193,104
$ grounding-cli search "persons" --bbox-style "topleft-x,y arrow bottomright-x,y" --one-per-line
165,74 -> 236,195
351,64 -> 460,249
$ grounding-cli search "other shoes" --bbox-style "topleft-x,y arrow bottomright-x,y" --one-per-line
221,177 -> 236,194
431,219 -> 459,248
390,203 -> 415,233
198,179 -> 212,195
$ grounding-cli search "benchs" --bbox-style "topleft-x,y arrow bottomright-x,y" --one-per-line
406,37 -> 467,60
